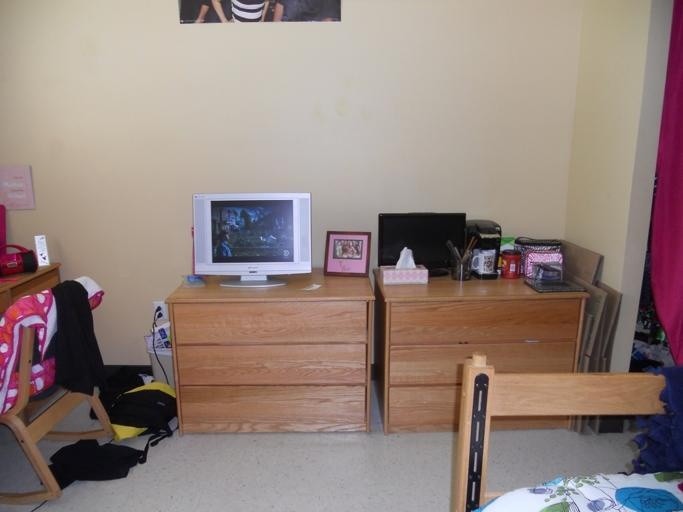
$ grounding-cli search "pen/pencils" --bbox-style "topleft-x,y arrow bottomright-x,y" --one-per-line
446,236 -> 479,277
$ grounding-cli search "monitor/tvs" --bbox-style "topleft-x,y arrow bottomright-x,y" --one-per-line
190,192 -> 312,289
377,212 -> 466,278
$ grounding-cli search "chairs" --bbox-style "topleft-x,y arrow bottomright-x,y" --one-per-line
0,275 -> 120,505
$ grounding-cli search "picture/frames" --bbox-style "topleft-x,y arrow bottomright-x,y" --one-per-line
323,230 -> 371,277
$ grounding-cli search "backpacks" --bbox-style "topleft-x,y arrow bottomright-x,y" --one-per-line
111,382 -> 177,428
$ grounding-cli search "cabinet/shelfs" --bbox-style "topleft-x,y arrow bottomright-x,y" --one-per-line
373,267 -> 586,434
165,269 -> 375,435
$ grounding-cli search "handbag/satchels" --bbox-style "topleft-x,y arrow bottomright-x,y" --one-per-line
90,375 -> 144,418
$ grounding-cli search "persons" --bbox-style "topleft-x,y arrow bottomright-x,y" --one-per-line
215,229 -> 231,257
210,1 -> 270,22
272,0 -> 336,22
335,240 -> 361,259
180,1 -> 210,23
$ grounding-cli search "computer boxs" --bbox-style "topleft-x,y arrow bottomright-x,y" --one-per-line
466,219 -> 502,280
